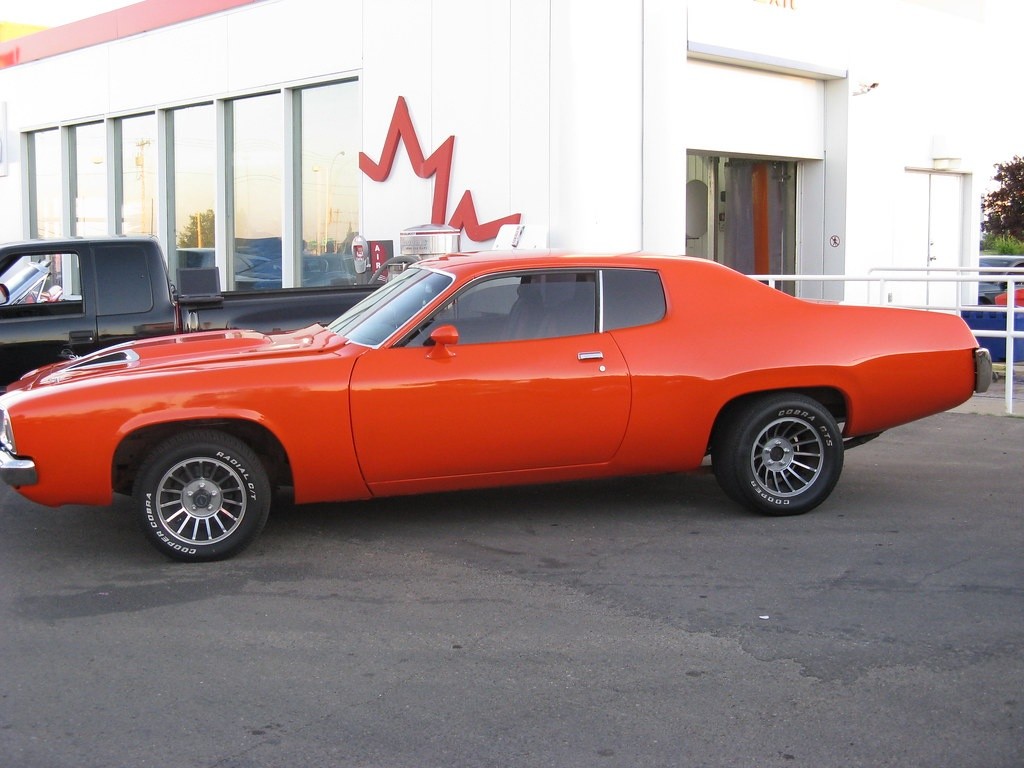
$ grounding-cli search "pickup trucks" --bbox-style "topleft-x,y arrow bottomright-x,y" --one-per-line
0,238 -> 391,392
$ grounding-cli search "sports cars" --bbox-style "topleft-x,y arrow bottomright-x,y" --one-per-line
0,253 -> 998,567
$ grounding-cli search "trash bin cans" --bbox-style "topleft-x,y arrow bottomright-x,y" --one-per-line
399,224 -> 461,260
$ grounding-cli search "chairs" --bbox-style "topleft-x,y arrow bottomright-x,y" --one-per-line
502,286 -> 544,339
